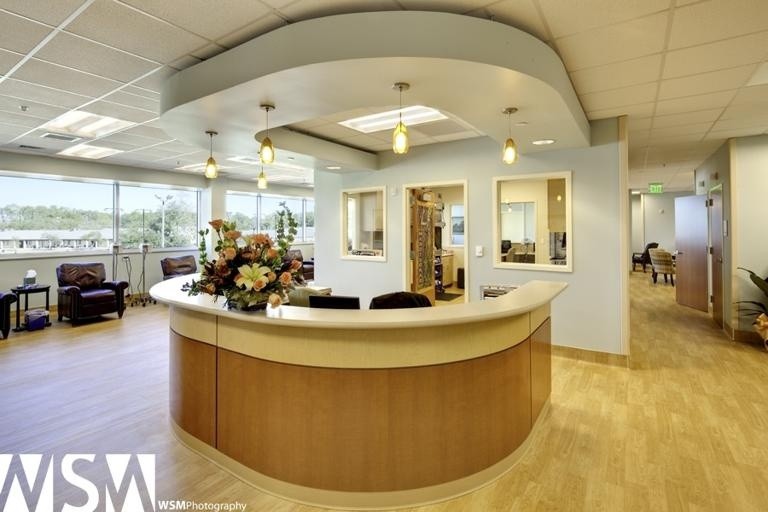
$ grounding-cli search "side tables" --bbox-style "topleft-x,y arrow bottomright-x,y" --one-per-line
10,285 -> 52,332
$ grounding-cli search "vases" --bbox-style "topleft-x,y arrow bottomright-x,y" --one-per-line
228,300 -> 266,311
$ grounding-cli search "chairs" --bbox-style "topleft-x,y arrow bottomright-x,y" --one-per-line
56,263 -> 128,327
631,242 -> 676,286
282,250 -> 314,280
369,292 -> 432,309
506,247 -> 516,263
0,293 -> 18,339
160,255 -> 196,280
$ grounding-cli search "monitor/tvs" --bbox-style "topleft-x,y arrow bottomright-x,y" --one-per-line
309,295 -> 361,309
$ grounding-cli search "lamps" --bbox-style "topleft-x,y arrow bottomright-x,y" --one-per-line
205,131 -> 217,179
257,152 -> 266,189
502,107 -> 518,165
392,83 -> 410,154
260,105 -> 275,164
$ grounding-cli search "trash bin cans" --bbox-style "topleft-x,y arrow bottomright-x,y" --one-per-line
26,309 -> 49,331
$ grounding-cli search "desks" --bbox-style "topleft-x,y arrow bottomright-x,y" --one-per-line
501,252 -> 535,263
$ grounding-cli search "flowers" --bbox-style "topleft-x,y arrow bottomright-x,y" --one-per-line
179,201 -> 303,311
